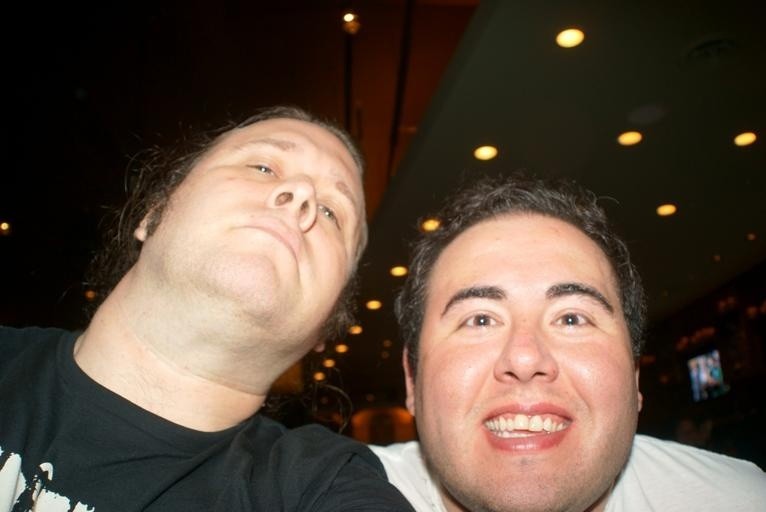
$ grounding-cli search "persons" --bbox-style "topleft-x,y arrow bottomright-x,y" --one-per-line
365,170 -> 766,510
687,352 -> 726,401
1,98 -> 417,511
660,403 -> 731,458
